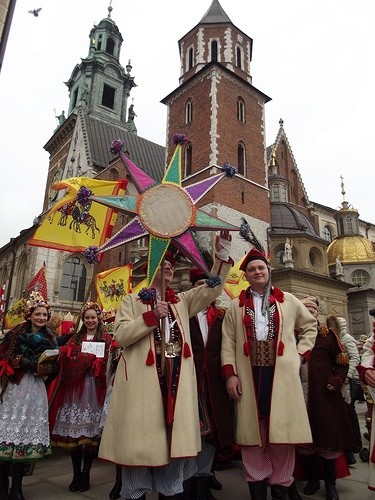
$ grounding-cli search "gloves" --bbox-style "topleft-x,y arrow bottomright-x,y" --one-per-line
20,357 -> 37,374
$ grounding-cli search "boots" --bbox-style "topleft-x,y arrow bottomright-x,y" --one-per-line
271,485 -> 287,500
180,477 -> 196,500
303,455 -> 320,495
249,484 -> 267,500
79,450 -> 94,492
110,464 -> 122,500
345,450 -> 356,465
125,490 -> 146,500
211,474 -> 222,490
288,485 -> 302,500
197,476 -> 215,500
69,450 -> 81,492
159,491 -> 176,500
323,458 -> 339,500
10,462 -> 25,500
0,463 -> 9,500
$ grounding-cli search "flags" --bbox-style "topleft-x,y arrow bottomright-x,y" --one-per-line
93,264 -> 133,312
26,176 -> 129,264
0,267 -> 48,330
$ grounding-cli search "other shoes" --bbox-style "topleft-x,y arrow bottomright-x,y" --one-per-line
363,432 -> 370,440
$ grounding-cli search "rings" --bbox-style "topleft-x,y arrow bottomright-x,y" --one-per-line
162,309 -> 164,313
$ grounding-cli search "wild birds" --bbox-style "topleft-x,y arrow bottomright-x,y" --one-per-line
27,6 -> 42,17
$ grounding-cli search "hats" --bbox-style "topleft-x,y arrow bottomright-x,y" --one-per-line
189,268 -> 209,286
300,295 -> 319,311
142,251 -> 175,274
239,248 -> 268,272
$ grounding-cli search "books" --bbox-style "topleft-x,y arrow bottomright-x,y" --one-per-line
32,348 -> 60,376
81,341 -> 105,358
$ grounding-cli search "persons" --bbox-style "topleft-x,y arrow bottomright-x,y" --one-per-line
109,268 -> 234,500
45,301 -> 120,493
128,104 -> 138,122
220,248 -> 319,500
54,110 -> 67,125
283,237 -> 293,261
0,290 -> 61,500
335,254 -> 343,275
285,294 -> 375,500
56,312 -> 76,346
99,229 -> 235,500
